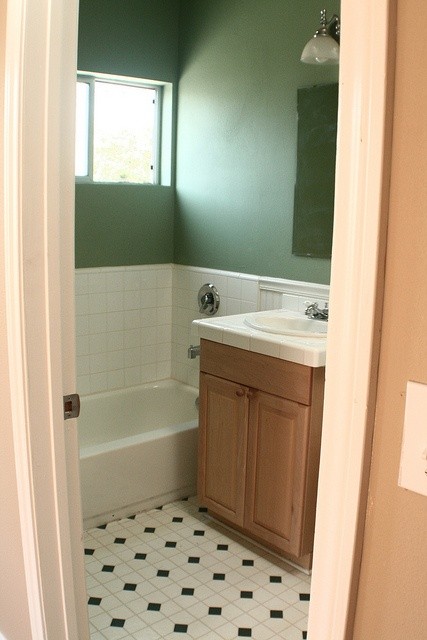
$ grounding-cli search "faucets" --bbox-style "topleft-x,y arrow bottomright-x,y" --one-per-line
305,302 -> 328,320
187,344 -> 200,361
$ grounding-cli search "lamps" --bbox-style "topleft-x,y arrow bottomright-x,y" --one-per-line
299,7 -> 339,66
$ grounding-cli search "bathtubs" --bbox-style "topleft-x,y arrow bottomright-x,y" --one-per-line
77,377 -> 199,529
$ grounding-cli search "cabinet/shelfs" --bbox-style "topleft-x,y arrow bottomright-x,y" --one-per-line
193,334 -> 325,577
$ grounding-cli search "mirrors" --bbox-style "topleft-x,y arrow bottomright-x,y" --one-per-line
290,81 -> 339,258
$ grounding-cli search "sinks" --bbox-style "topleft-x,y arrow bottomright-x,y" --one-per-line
244,312 -> 327,339
